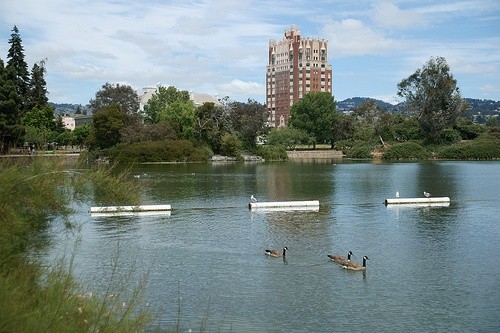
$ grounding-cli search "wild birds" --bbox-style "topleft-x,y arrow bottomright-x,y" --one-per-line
340,256 -> 370,270
423,191 -> 431,198
326,251 -> 354,265
250,195 -> 257,203
264,246 -> 289,257
395,191 -> 399,198
